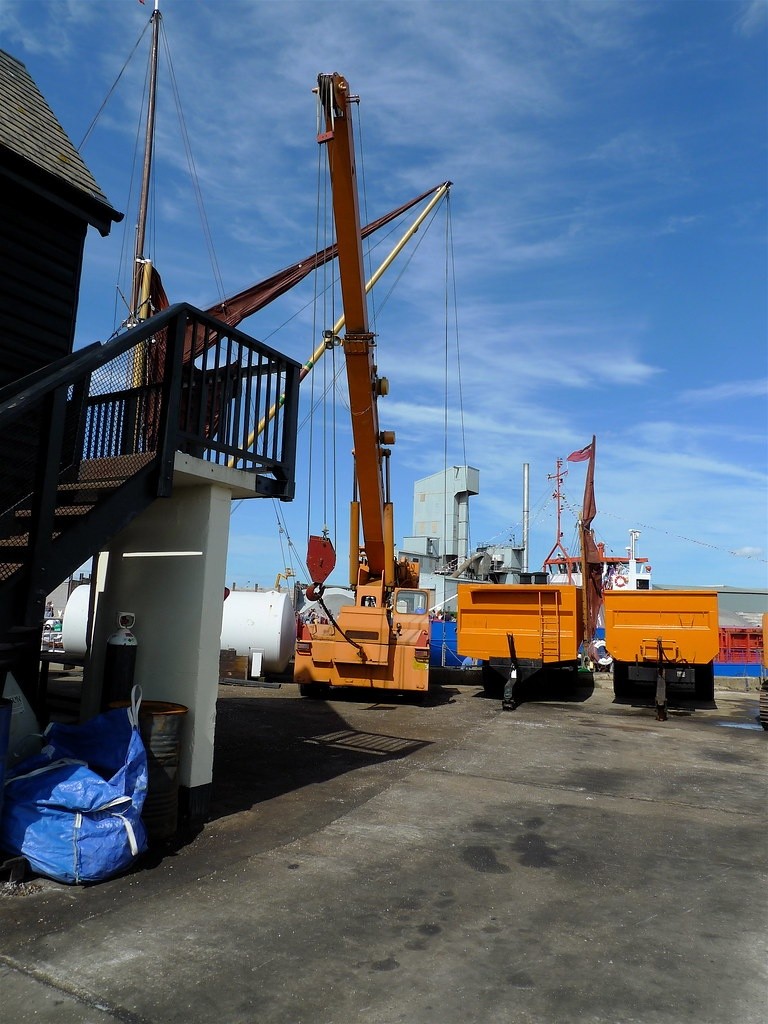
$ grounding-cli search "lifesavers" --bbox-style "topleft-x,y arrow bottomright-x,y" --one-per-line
594,640 -> 613,665
615,576 -> 627,587
588,641 -> 601,662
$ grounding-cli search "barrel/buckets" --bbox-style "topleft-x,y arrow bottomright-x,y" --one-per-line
109,700 -> 189,837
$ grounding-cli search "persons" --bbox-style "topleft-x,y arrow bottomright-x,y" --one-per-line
429,609 -> 456,622
44,601 -> 54,617
555,553 -> 563,574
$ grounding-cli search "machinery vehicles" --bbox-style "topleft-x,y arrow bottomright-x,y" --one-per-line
273,73 -> 451,702
